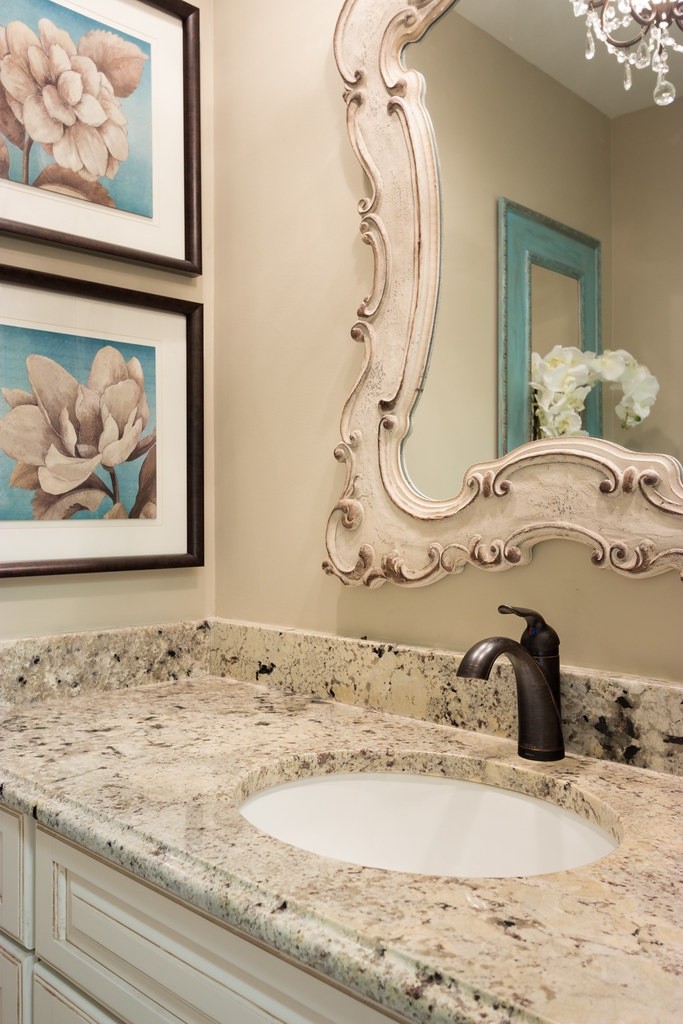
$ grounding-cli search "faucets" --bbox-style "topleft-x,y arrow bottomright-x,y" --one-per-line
456,605 -> 566,762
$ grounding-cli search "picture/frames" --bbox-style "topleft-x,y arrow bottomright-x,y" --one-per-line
0,263 -> 205,579
0,0 -> 203,279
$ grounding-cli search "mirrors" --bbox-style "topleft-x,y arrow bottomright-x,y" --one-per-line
496,196 -> 604,458
319,0 -> 683,591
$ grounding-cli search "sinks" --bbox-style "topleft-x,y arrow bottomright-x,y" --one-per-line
237,744 -> 626,879
